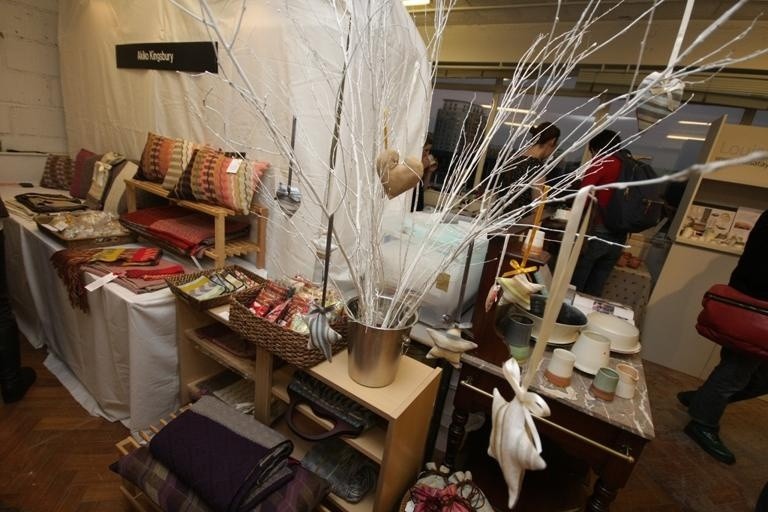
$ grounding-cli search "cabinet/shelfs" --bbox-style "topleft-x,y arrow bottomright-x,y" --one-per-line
125,178 -> 268,271
174,294 -> 444,512
640,114 -> 768,407
115,401 -> 328,511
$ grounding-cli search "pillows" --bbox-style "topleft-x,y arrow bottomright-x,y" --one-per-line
139,132 -> 176,183
161,138 -> 222,190
166,148 -> 271,216
109,439 -> 333,512
39,146 -> 152,218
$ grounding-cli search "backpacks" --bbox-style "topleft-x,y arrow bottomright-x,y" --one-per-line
597,148 -> 664,233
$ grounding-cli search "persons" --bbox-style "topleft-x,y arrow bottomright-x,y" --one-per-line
411,136 -> 437,211
570,129 -> 635,297
491,123 -> 561,235
677,208 -> 768,464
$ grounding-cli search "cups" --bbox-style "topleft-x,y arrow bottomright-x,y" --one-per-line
615,364 -> 640,400
718,212 -> 731,229
617,252 -> 640,270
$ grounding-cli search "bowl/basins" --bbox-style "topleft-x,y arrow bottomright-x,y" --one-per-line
512,294 -> 589,338
586,312 -> 640,349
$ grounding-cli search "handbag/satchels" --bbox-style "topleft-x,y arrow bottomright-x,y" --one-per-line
695,284 -> 767,361
287,370 -> 387,442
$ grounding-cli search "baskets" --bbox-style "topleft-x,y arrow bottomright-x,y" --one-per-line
164,264 -> 269,313
228,282 -> 347,371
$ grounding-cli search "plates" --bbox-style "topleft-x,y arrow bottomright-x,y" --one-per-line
530,332 -> 580,346
581,328 -> 642,354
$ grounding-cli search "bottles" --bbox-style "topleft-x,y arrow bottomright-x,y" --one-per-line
505,314 -> 535,366
568,330 -> 611,375
709,212 -> 720,229
547,348 -> 575,387
590,368 -> 619,402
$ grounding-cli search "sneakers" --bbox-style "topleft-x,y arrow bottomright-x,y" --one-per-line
2,366 -> 35,404
678,391 -> 698,406
684,419 -> 735,465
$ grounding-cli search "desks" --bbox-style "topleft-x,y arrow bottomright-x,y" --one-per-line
444,294 -> 655,512
1,194 -> 268,434
599,260 -> 652,333
